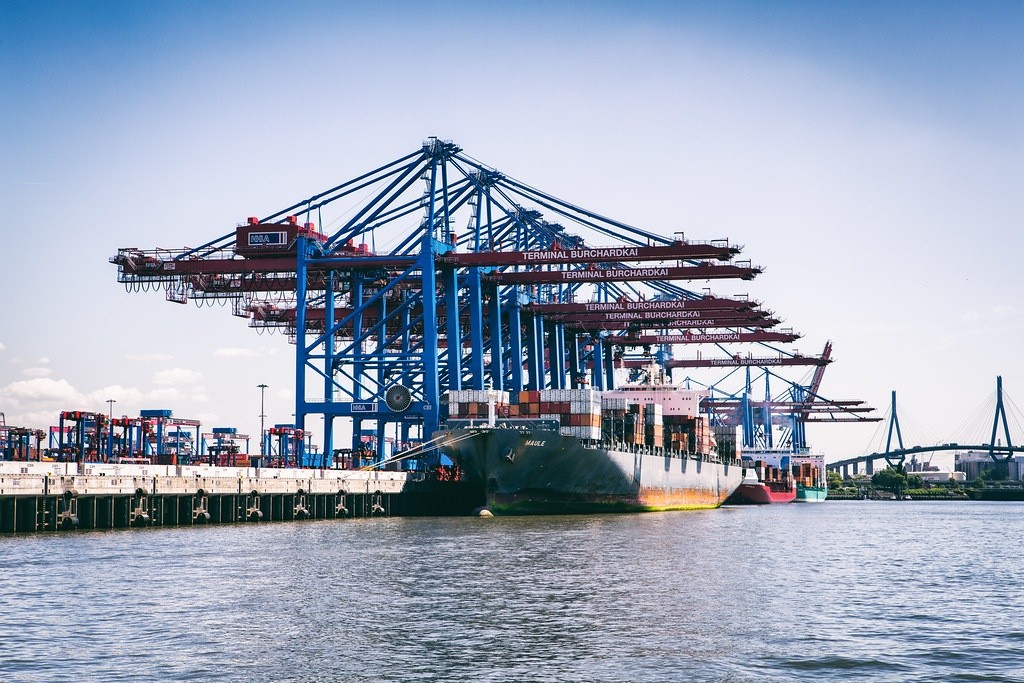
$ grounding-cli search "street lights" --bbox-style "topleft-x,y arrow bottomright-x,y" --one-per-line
257,384 -> 269,468
106,400 -> 116,459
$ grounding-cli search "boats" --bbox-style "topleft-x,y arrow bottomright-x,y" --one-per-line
432,377 -> 746,516
791,482 -> 828,503
738,479 -> 796,504
